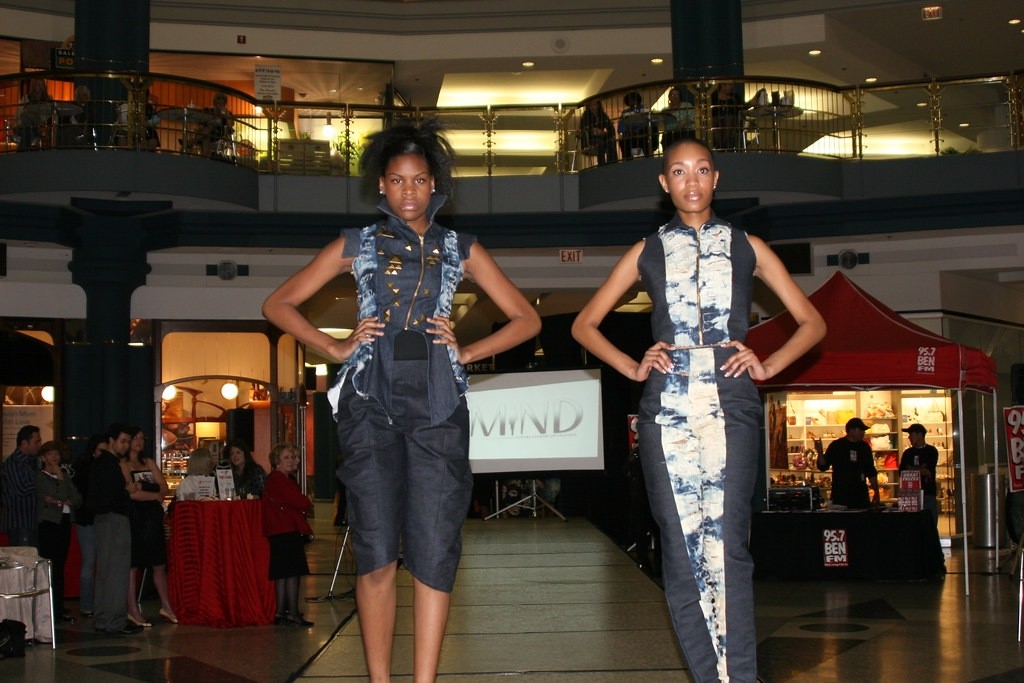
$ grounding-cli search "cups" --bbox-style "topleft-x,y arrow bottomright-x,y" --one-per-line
187,104 -> 196,108
225,488 -> 235,500
633,105 -> 644,114
239,488 -> 246,499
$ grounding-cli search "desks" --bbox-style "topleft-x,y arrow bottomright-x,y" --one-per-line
0,498 -> 277,627
29,102 -> 85,150
757,507 -> 946,579
747,103 -> 804,154
622,113 -> 684,158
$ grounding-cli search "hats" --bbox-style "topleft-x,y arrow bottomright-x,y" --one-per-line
846,418 -> 869,430
902,424 -> 926,434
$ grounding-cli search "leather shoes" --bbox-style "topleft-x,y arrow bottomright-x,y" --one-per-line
95,628 -> 106,632
106,625 -> 144,634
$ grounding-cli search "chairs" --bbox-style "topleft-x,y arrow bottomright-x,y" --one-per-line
197,106 -> 237,162
108,102 -> 134,150
1,557 -> 56,650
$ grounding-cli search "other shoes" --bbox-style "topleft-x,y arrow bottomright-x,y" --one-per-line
82,612 -> 93,618
59,609 -> 76,622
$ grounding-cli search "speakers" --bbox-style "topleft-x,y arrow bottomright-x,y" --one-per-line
228,408 -> 255,453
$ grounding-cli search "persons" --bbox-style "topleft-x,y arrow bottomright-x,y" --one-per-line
36,440 -> 74,624
168,447 -> 219,522
0,425 -> 41,546
571,138 -> 827,682
579,98 -> 618,165
768,394 -> 784,453
12,79 -> 55,151
711,83 -> 766,151
262,444 -> 316,627
70,423 -> 144,634
660,88 -> 696,154
263,98 -> 542,683
179,92 -> 234,149
58,85 -> 91,123
118,426 -> 178,626
618,92 -> 659,161
811,418 -> 880,510
213,437 -> 267,500
120,82 -> 161,148
899,424 -> 939,528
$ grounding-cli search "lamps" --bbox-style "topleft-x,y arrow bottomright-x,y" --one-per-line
162,385 -> 178,400
221,379 -> 238,400
40,385 -> 55,403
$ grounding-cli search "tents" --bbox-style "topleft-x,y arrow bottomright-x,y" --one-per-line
743,270 -> 999,596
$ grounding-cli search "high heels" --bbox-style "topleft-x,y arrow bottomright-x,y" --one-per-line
275,611 -> 287,624
159,608 -> 178,623
286,611 -> 314,627
128,613 -> 152,627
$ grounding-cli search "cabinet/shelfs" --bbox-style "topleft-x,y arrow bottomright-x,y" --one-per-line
783,420 -> 956,517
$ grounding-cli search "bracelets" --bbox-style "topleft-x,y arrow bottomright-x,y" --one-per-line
57,500 -> 61,506
